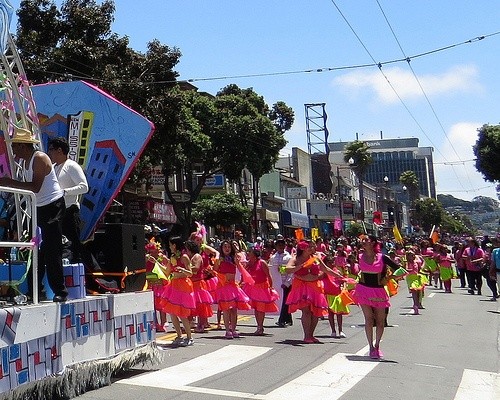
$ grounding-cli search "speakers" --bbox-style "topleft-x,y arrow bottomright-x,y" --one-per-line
104,222 -> 146,291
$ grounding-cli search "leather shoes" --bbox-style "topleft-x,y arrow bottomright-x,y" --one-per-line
23,294 -> 44,301
53,295 -> 66,302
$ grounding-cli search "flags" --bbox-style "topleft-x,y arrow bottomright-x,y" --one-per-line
295,217 -> 438,245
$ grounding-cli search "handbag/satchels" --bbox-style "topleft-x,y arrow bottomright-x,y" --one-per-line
489,261 -> 496,281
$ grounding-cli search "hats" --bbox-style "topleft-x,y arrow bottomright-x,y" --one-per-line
4,128 -> 40,143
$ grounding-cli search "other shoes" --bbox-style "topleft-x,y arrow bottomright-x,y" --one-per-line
331,332 -> 336,337
496,293 -> 499,298
303,338 -> 314,343
339,332 -> 346,337
275,322 -> 292,328
254,326 -> 264,335
478,291 -> 481,295
375,346 -> 383,357
468,289 -> 474,295
414,305 -> 418,314
445,289 -> 451,292
311,336 -> 319,342
419,305 -> 425,309
155,320 -> 239,346
434,285 -> 437,288
370,350 -> 379,359
440,286 -> 442,289
461,285 -> 465,288
491,297 -> 496,301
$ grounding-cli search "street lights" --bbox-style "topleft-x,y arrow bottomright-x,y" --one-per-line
393,186 -> 408,226
336,158 -> 356,232
376,176 -> 389,213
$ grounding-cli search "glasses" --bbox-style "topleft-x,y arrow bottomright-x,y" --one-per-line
48,147 -> 58,151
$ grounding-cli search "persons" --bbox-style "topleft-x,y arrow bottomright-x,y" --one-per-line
46,136 -> 102,297
0,128 -> 67,302
144,225 -> 500,359
